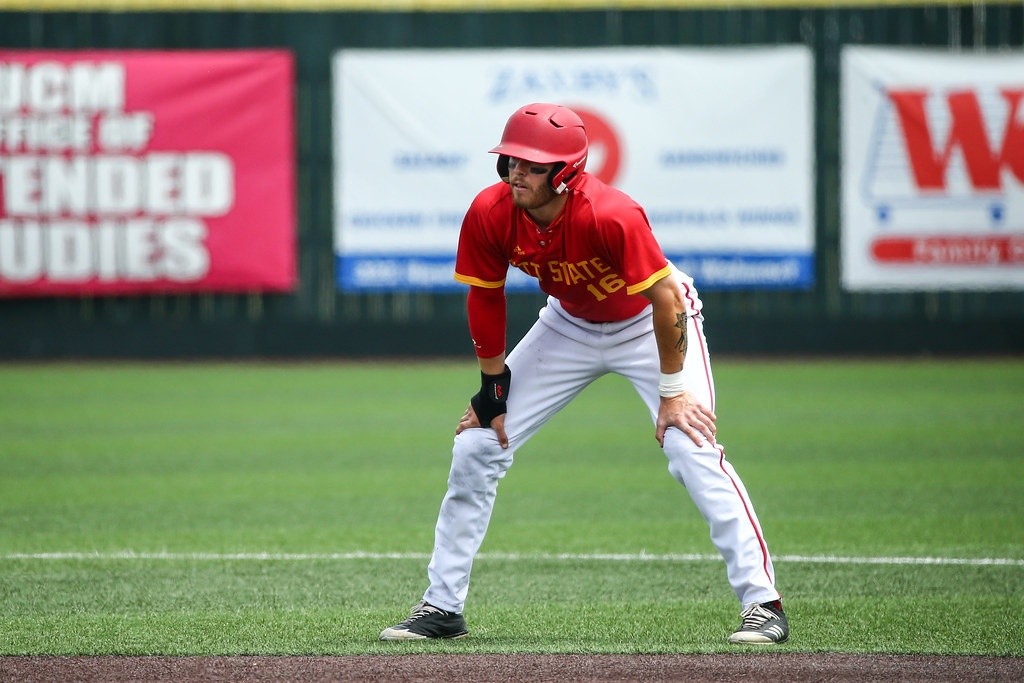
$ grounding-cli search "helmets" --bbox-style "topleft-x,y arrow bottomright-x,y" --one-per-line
487,102 -> 589,196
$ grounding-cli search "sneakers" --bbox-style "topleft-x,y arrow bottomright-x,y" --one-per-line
727,602 -> 790,646
378,599 -> 469,641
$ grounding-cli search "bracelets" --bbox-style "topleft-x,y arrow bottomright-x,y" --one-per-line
658,370 -> 685,398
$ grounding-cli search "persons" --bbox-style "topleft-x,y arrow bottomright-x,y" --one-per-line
380,102 -> 789,644
